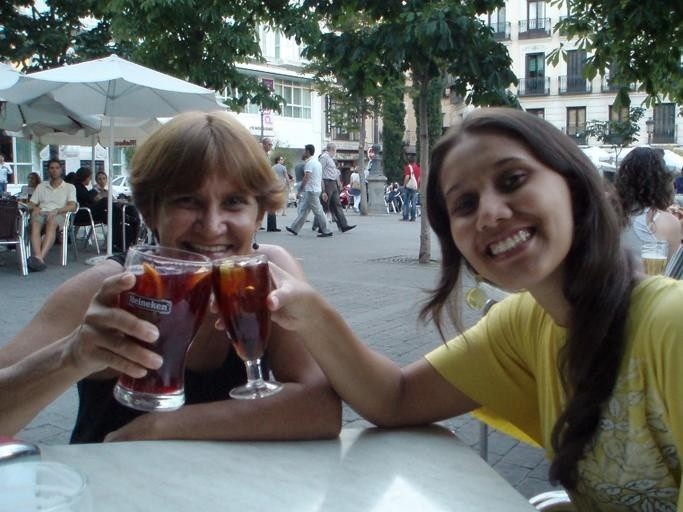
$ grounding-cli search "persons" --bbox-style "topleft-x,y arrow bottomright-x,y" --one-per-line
612,146 -> 683,259
207,107 -> 683,512
256,137 -> 421,238
0,154 -> 140,271
0,110 -> 343,444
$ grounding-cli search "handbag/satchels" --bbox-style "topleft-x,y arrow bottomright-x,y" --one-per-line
352,183 -> 361,190
287,187 -> 296,203
405,177 -> 417,191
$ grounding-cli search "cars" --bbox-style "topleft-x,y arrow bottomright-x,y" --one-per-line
570,143 -> 683,194
104,176 -> 134,204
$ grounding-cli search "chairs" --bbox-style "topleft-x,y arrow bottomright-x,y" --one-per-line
1,182 -> 148,282
461,283 -> 545,462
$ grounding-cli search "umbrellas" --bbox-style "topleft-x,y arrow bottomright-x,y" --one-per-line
39,117 -> 163,187
0,53 -> 230,257
0,63 -> 103,138
599,147 -> 683,178
578,144 -> 616,173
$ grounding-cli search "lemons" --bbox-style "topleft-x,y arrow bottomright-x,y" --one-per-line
143,262 -> 162,299
220,263 -> 246,293
186,271 -> 212,289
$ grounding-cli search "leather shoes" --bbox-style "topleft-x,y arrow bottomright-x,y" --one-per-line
268,228 -> 281,232
286,227 -> 297,235
317,233 -> 333,237
341,225 -> 356,232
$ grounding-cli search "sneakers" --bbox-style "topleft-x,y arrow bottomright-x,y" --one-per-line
27,256 -> 46,271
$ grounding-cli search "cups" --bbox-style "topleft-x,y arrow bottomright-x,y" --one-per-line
110,245 -> 214,415
641,239 -> 669,276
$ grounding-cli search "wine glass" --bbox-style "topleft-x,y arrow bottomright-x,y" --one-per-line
212,254 -> 284,401
